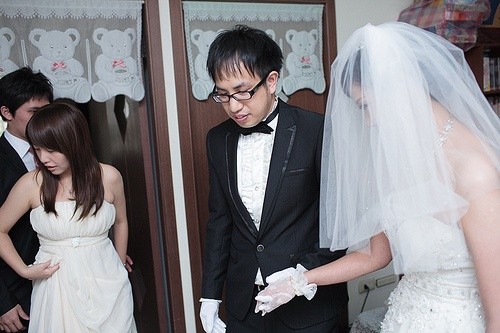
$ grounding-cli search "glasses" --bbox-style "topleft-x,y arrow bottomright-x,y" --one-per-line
213,76 -> 266,103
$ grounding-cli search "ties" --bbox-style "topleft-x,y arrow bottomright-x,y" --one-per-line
28,148 -> 39,170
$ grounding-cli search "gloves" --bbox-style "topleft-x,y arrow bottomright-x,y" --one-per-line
199,298 -> 226,333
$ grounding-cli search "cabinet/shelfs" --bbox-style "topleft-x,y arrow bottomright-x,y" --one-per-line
465,23 -> 500,97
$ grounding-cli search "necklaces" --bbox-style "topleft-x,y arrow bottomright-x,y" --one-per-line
61,179 -> 72,195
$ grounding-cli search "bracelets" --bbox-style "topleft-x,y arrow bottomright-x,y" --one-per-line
123,263 -> 126,267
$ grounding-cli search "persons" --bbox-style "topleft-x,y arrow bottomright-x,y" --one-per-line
199,25 -> 348,333
320,22 -> 500,333
0,66 -> 137,333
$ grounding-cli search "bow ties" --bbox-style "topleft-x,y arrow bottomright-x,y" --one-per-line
239,106 -> 279,136
